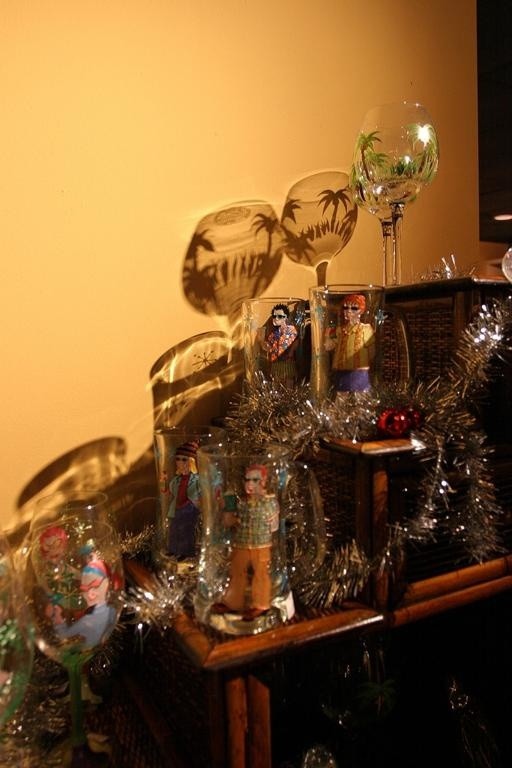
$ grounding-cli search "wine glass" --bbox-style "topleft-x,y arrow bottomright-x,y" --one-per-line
347,100 -> 439,289
17,489 -> 128,768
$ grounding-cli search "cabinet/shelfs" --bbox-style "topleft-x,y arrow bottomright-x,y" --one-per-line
0,270 -> 509,766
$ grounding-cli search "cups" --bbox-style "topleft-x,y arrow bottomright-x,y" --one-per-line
153,425 -> 295,638
242,297 -> 310,405
308,281 -> 385,412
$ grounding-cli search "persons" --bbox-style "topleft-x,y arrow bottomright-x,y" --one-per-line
162,440 -> 216,559
37,526 -> 82,584
46,558 -> 116,651
323,293 -> 378,398
211,461 -> 280,622
262,304 -> 298,387
